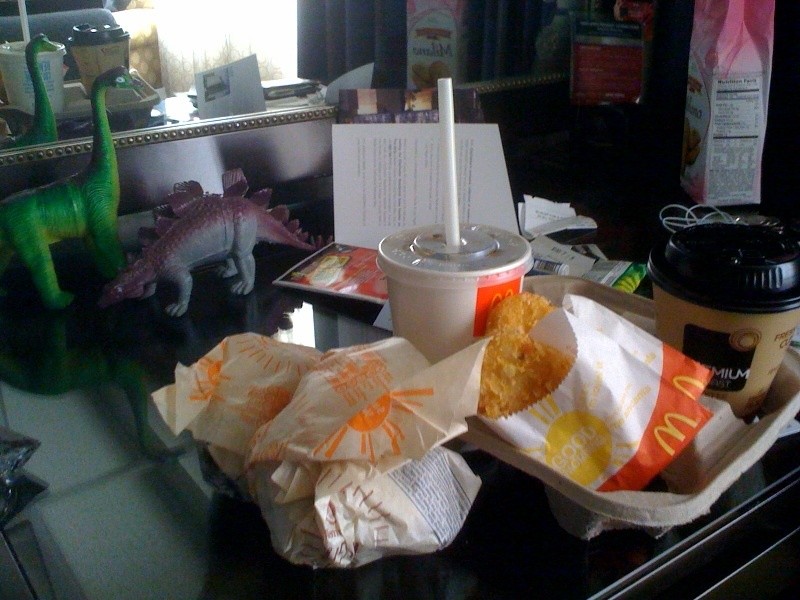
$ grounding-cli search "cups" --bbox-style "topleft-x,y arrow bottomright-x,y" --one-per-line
0,41 -> 67,116
646,224 -> 800,420
376,223 -> 534,365
67,23 -> 131,91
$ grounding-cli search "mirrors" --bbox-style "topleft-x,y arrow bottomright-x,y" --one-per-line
0,0 -> 590,166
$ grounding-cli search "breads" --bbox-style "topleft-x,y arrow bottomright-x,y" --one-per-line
412,61 -> 449,88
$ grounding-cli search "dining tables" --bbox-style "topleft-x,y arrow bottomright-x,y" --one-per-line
0,200 -> 800,600
